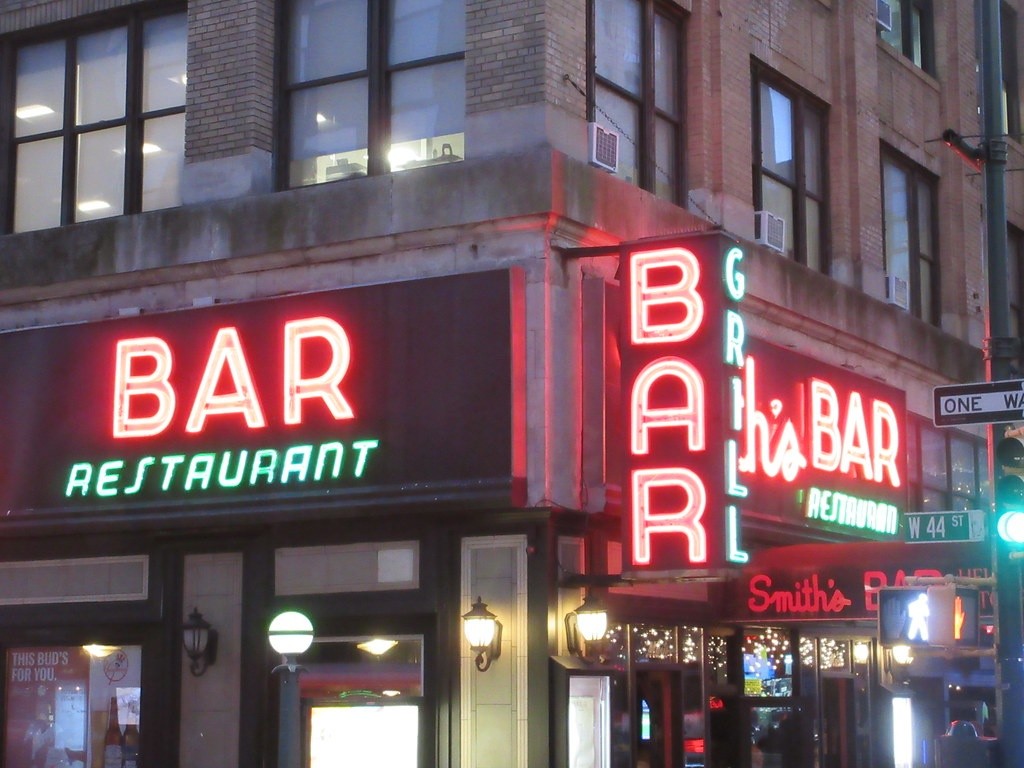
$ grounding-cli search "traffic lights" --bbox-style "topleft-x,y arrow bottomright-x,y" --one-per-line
989,426 -> 1024,569
876,582 -> 991,657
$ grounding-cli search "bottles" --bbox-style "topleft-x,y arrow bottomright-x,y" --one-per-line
103,697 -> 123,768
122,702 -> 139,768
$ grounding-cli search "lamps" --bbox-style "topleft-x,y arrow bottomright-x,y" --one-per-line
854,641 -> 870,678
461,596 -> 503,672
884,644 -> 915,683
564,589 -> 609,666
180,607 -> 218,677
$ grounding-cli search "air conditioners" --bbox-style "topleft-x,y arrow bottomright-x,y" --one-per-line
885,275 -> 909,309
755,210 -> 784,254
876,0 -> 892,34
587,122 -> 619,173
